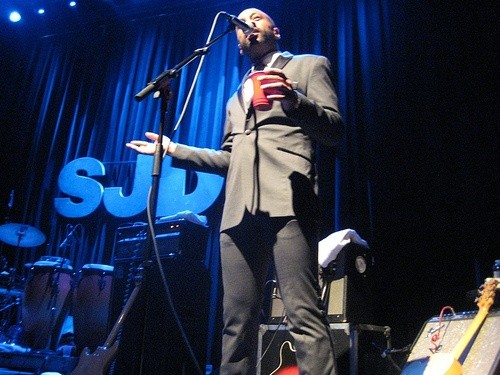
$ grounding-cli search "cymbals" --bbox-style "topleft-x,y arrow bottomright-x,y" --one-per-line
0,223 -> 46,247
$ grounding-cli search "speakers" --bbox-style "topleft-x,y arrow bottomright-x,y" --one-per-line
397,308 -> 500,375
101,255 -> 224,375
267,244 -> 375,325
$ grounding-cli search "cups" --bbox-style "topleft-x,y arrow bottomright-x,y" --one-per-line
250,70 -> 275,110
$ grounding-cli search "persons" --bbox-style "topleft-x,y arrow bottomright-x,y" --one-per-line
126,8 -> 345,375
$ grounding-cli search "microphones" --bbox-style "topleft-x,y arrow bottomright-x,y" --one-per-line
221,11 -> 255,35
7,189 -> 15,208
430,323 -> 445,333
372,343 -> 387,359
59,224 -> 80,248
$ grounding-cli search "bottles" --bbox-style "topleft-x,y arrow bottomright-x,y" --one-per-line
493,260 -> 500,278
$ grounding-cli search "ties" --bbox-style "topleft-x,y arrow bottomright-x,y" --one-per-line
254,61 -> 266,70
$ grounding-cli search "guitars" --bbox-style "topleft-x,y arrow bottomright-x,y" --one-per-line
398,278 -> 498,375
68,265 -> 145,375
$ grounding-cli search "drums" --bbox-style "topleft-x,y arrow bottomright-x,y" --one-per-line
73,264 -> 114,356
21,261 -> 75,354
